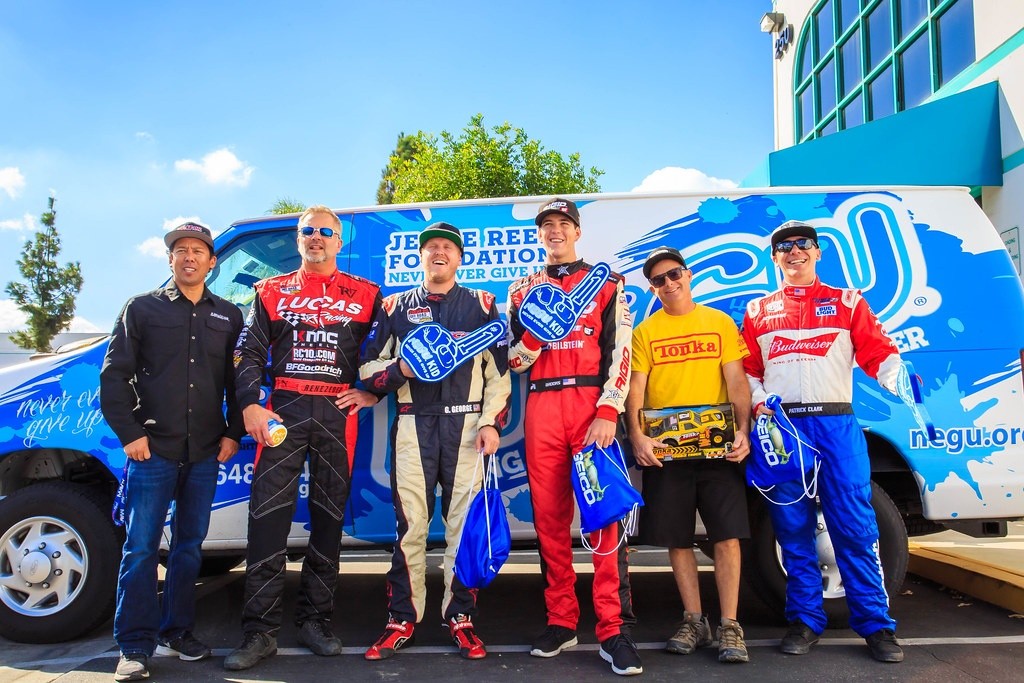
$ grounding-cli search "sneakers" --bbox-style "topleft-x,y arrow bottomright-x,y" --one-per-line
716,617 -> 749,662
599,633 -> 643,675
225,631 -> 277,669
665,611 -> 712,654
363,613 -> 415,659
780,620 -> 819,654
530,624 -> 577,657
298,622 -> 342,656
155,634 -> 211,662
866,628 -> 904,661
442,612 -> 487,659
114,652 -> 151,681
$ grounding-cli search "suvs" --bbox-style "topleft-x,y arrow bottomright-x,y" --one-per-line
647,407 -> 731,450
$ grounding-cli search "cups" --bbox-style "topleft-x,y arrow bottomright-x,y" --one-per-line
264,419 -> 287,448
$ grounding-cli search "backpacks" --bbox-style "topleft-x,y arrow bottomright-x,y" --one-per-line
571,438 -> 644,555
453,448 -> 512,589
745,394 -> 823,505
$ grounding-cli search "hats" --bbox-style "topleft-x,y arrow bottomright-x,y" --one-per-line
643,246 -> 688,281
164,221 -> 213,247
535,197 -> 580,226
771,220 -> 818,248
419,222 -> 463,249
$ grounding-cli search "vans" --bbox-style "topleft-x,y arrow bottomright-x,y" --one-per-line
0,186 -> 1022,645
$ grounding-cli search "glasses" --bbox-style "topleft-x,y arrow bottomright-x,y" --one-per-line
775,238 -> 813,252
647,265 -> 686,288
297,226 -> 340,239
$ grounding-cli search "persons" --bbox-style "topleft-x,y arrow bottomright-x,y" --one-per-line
224,205 -> 383,669
357,222 -> 513,660
741,221 -> 903,661
100,222 -> 244,683
505,198 -> 643,675
627,248 -> 751,662
